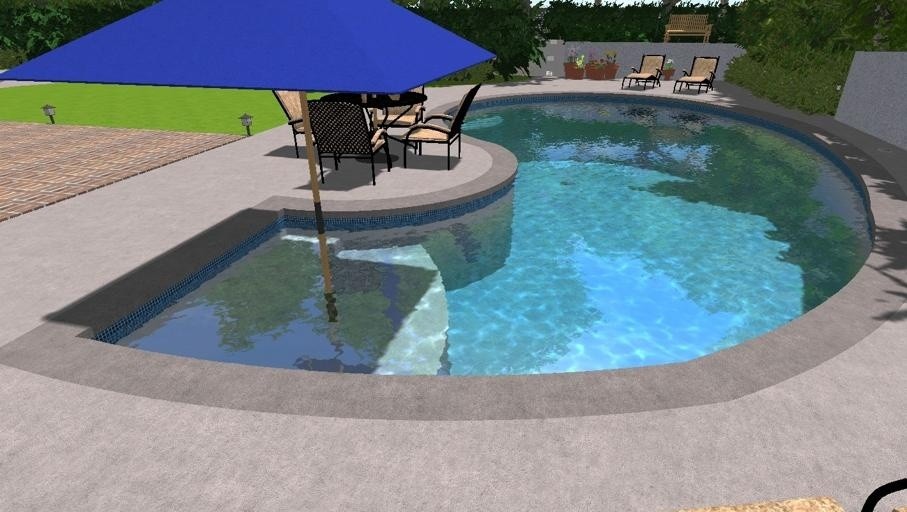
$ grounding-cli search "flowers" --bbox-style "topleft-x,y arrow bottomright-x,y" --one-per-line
564,43 -> 617,71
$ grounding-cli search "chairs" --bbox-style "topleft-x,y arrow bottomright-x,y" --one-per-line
273,84 -> 484,187
621,53 -> 721,95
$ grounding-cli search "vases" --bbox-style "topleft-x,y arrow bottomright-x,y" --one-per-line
564,61 -> 618,81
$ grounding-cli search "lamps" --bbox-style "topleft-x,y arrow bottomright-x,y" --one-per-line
41,104 -> 57,125
239,111 -> 255,136
